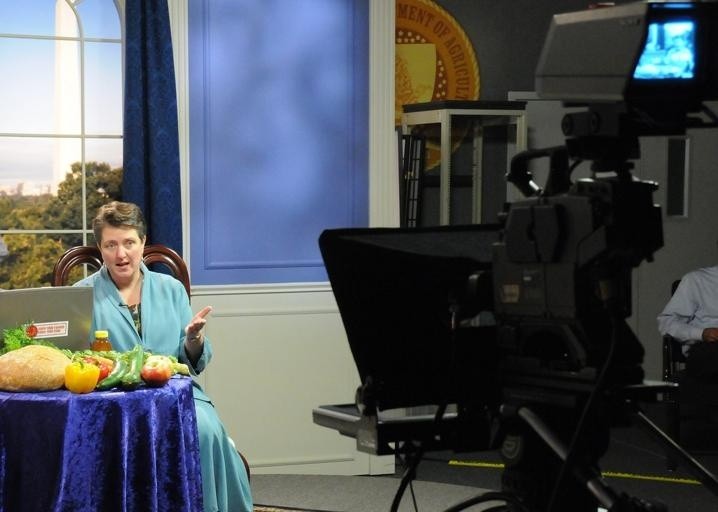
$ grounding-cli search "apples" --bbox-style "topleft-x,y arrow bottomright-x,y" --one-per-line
147,355 -> 174,373
141,361 -> 171,386
84,358 -> 109,382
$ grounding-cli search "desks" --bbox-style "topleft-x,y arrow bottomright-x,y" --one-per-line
0,366 -> 204,511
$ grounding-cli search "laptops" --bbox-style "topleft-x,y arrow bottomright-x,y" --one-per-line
0,287 -> 94,352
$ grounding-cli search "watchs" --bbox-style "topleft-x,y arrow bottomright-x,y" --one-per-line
188,333 -> 202,343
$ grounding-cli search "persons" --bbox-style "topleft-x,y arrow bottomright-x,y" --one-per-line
664,35 -> 694,73
71,201 -> 254,512
656,264 -> 718,448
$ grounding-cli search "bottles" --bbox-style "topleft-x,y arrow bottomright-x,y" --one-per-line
90,330 -> 112,352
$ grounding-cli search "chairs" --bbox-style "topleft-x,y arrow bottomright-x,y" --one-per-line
662,274 -> 689,429
50,244 -> 252,487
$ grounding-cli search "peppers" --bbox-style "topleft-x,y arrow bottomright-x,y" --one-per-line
122,344 -> 143,388
96,354 -> 128,390
65,361 -> 100,394
